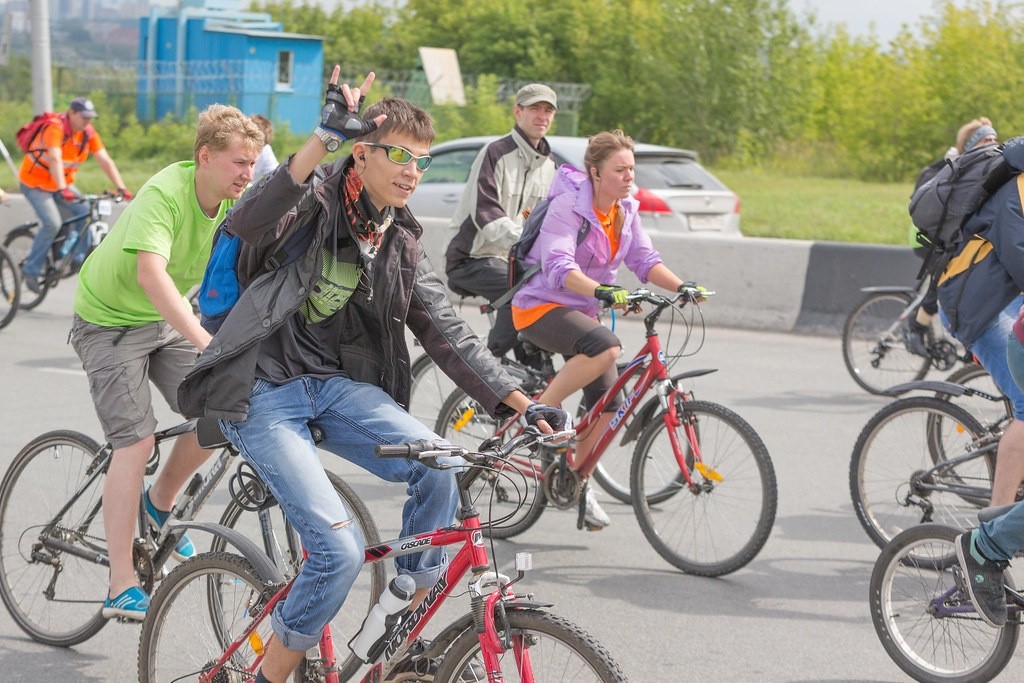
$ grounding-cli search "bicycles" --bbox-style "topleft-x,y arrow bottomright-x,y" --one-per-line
925,358 -> 1023,510
134,427 -> 629,683
0,243 -> 19,332
842,282 -> 984,397
402,281 -> 779,577
869,525 -> 1024,682
848,381 -> 1024,569
0,188 -> 132,310
0,354 -> 387,679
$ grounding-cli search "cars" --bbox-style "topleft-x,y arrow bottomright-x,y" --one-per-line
405,134 -> 742,237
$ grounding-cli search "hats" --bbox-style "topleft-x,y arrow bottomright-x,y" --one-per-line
71,97 -> 98,119
515,83 -> 558,110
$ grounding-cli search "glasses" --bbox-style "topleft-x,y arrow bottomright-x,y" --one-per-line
357,141 -> 433,172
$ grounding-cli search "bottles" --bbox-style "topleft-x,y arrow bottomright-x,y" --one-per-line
59,231 -> 79,257
348,574 -> 416,664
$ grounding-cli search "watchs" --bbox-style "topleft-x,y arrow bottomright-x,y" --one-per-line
313,127 -> 341,153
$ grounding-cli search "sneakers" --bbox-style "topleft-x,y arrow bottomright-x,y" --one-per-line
954,528 -> 1009,629
101,582 -> 150,620
379,633 -> 487,683
574,483 -> 611,528
143,482 -> 196,562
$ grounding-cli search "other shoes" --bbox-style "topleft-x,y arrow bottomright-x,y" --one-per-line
900,320 -> 930,357
23,270 -> 40,294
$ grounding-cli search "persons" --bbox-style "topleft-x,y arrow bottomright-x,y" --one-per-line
66,103 -> 263,617
252,116 -> 278,185
905,115 -> 999,357
444,84 -> 558,364
19,100 -> 134,293
512,133 -> 706,528
935,168 -> 1024,506
177,63 -> 574,683
954,305 -> 1024,628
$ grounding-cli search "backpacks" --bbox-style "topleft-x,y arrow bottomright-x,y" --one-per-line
16,111 -> 71,154
479,193 -> 590,314
908,143 -> 1019,281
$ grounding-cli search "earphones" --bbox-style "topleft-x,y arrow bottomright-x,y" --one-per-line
359,154 -> 365,161
596,171 -> 600,177
